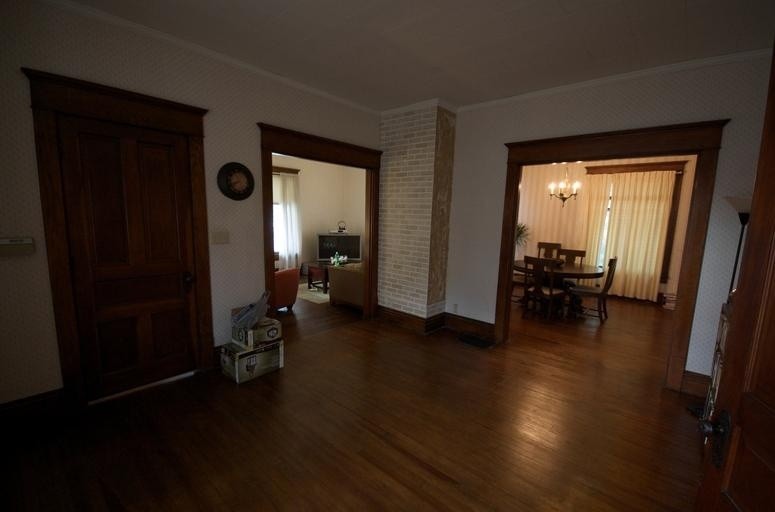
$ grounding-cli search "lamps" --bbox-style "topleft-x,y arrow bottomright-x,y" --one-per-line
721,196 -> 752,301
547,167 -> 580,207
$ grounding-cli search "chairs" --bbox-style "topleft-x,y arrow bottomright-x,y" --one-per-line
275,267 -> 301,311
522,256 -> 566,320
559,248 -> 586,264
538,242 -> 561,261
569,256 -> 618,319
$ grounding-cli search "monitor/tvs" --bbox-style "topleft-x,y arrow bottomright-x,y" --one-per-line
316,234 -> 362,262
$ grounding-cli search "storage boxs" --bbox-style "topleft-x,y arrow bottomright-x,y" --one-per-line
230,307 -> 282,344
220,340 -> 284,384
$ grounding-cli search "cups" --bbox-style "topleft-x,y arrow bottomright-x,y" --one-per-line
331,255 -> 347,264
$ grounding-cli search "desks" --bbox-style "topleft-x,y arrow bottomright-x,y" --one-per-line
514,261 -> 604,316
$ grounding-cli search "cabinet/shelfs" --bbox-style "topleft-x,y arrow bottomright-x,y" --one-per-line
703,303 -> 757,425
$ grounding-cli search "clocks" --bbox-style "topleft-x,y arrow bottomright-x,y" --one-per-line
216,161 -> 255,200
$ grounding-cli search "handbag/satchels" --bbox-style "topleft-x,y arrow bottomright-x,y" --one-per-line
233,287 -> 272,329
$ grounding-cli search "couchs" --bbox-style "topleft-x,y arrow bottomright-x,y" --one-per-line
327,262 -> 364,313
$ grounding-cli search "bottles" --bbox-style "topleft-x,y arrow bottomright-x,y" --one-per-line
335,252 -> 340,265
